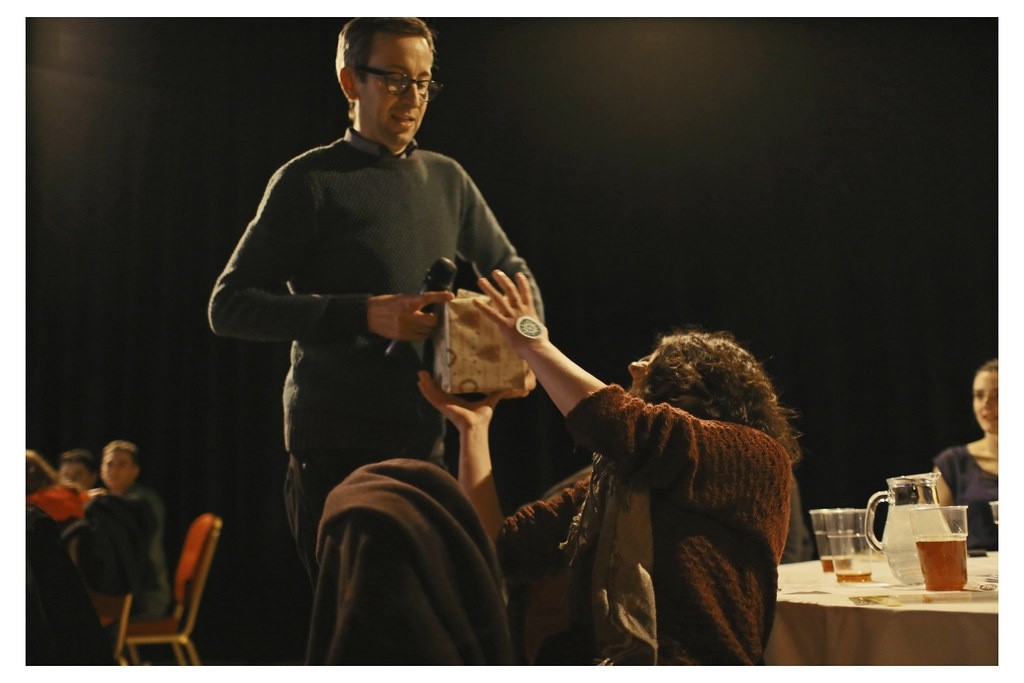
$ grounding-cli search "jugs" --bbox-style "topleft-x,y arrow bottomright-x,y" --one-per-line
865,472 -> 943,588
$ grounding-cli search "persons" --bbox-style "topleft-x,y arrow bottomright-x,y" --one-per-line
417,270 -> 804,666
208,17 -> 546,598
933,357 -> 998,559
26,440 -> 171,666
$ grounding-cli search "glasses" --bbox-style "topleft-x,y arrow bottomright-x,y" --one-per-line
354,67 -> 443,102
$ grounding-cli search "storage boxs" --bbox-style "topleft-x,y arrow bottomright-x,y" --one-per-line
431,288 -> 536,393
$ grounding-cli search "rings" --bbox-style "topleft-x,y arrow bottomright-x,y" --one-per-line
511,298 -> 523,304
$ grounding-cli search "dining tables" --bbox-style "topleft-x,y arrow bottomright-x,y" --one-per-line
763,551 -> 998,665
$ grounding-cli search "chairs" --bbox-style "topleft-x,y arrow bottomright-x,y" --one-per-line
59,521 -> 132,666
125,513 -> 222,666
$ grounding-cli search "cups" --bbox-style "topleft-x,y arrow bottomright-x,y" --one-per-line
823,510 -> 875,583
908,505 -> 968,591
989,501 -> 998,525
809,508 -> 856,573
